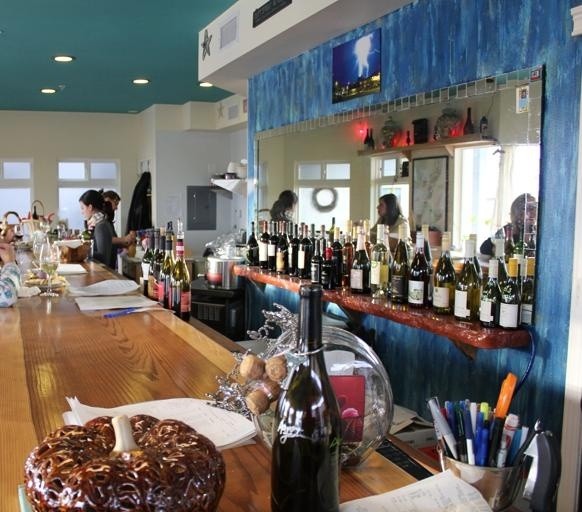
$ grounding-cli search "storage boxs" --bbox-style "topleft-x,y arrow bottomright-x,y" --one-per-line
385,402 -> 440,459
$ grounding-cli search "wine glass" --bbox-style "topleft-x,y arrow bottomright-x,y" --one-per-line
26,232 -> 61,299
15,225 -> 24,242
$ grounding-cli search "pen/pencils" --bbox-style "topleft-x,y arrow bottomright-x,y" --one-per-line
104,308 -> 135,318
425,372 -> 544,468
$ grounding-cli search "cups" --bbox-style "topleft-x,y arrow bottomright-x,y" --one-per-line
222,158 -> 248,180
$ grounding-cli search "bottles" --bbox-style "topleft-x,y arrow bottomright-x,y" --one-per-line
455,238 -> 480,321
479,259 -> 499,327
32,206 -> 38,219
479,112 -> 488,134
406,230 -> 430,307
432,107 -> 461,140
521,257 -> 533,326
134,219 -> 192,319
248,216 -> 370,292
389,225 -> 408,299
358,217 -> 533,273
498,257 -> 522,330
363,127 -> 375,151
462,107 -> 474,136
433,235 -> 454,313
369,223 -> 388,298
269,285 -> 343,511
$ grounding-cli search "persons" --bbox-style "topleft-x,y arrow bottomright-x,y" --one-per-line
370,193 -> 410,241
480,193 -> 538,257
99,191 -> 135,269
270,190 -> 298,223
0,243 -> 21,308
79,190 -> 114,267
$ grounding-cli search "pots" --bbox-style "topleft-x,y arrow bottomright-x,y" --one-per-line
207,256 -> 245,291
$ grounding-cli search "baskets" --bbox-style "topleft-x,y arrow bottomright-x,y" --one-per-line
21,200 -> 51,242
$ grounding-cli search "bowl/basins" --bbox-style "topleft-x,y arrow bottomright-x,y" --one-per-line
435,448 -> 534,512
55,241 -> 92,261
